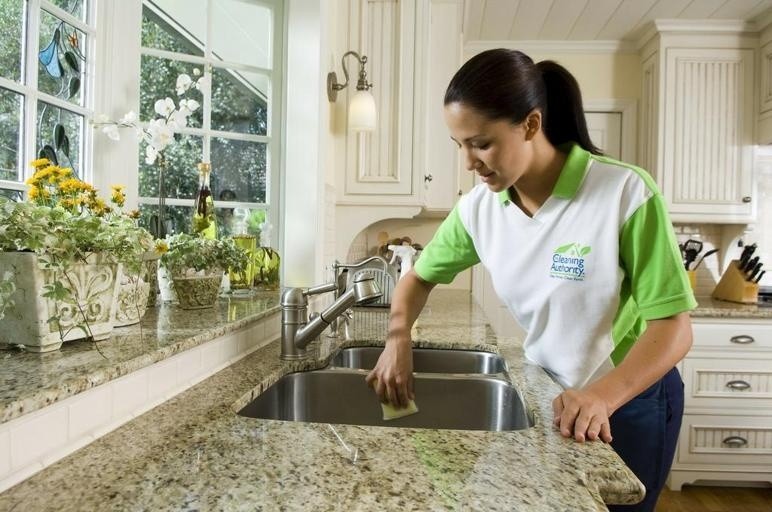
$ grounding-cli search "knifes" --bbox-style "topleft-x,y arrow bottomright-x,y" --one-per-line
738,243 -> 766,284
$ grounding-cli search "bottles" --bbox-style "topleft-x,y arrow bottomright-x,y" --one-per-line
254,223 -> 280,293
192,163 -> 219,240
229,207 -> 257,297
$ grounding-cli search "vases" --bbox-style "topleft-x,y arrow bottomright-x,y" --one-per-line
158,261 -> 180,301
114,250 -> 166,329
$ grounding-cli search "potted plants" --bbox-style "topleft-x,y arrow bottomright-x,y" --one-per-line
0,196 -> 127,352
159,233 -> 254,310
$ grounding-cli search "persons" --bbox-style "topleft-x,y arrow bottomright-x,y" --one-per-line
365,49 -> 695,511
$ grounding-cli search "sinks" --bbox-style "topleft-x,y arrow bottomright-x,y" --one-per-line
315,338 -> 509,376
228,363 -> 541,433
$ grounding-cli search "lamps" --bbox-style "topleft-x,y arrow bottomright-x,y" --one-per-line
327,51 -> 378,131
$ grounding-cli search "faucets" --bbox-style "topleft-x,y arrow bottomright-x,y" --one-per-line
281,274 -> 381,361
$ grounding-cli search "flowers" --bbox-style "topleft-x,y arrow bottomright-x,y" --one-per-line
89,66 -> 206,242
20,157 -> 169,252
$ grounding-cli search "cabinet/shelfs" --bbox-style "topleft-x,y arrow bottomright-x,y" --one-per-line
664,316 -> 772,492
756,24 -> 772,146
638,24 -> 754,225
326,2 -> 433,211
421,17 -> 463,211
